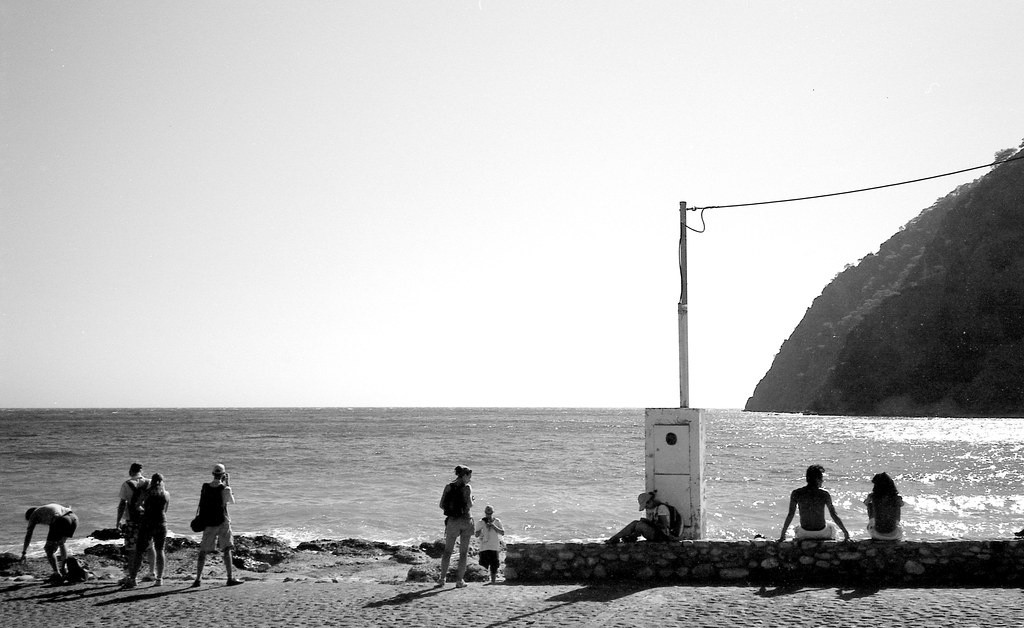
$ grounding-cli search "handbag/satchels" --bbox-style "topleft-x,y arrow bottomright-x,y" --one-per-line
191,516 -> 204,532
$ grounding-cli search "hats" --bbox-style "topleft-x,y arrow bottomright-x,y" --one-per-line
152,473 -> 163,481
638,493 -> 652,511
213,464 -> 224,474
485,505 -> 493,514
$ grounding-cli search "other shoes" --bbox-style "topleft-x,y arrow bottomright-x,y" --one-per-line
227,578 -> 246,586
45,573 -> 61,584
156,577 -> 163,586
146,572 -> 155,579
119,578 -> 137,591
456,579 -> 467,587
437,577 -> 446,586
604,536 -> 620,544
621,535 -> 636,543
1015,529 -> 1024,536
191,580 -> 201,587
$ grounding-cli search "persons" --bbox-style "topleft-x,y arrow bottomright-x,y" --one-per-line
191,463 -> 244,587
780,464 -> 850,540
116,463 -> 157,581
863,472 -> 905,540
474,506 -> 504,582
438,466 -> 473,588
606,492 -> 683,544
121,473 -> 170,588
21,503 -> 78,577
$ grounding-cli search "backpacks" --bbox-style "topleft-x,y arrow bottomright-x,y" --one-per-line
656,503 -> 684,536
60,556 -> 94,581
443,483 -> 465,517
125,479 -> 149,522
